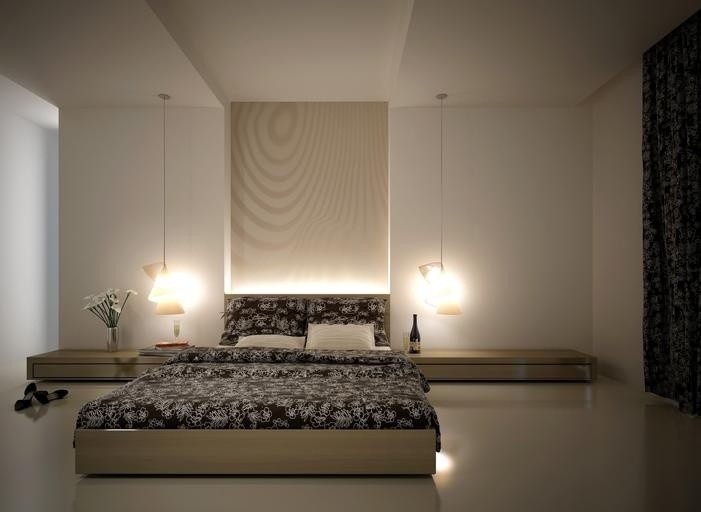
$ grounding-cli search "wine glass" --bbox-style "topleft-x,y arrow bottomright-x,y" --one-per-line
174,319 -> 181,343
402,332 -> 410,357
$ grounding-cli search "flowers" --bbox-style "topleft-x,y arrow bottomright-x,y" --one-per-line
79,288 -> 136,327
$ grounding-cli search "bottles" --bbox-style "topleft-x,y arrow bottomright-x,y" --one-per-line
409,314 -> 421,354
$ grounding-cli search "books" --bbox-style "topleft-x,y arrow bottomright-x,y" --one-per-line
137,339 -> 195,356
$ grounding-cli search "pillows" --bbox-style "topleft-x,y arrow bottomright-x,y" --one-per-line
220,297 -> 390,350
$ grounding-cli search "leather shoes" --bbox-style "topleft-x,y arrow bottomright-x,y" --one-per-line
34,389 -> 68,404
15,383 -> 36,411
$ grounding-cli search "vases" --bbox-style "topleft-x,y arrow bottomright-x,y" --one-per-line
106,326 -> 119,352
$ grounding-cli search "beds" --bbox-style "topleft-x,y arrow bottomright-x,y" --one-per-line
75,295 -> 436,475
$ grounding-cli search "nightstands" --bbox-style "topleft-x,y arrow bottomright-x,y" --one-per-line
27,350 -> 171,381
405,349 -> 598,382
